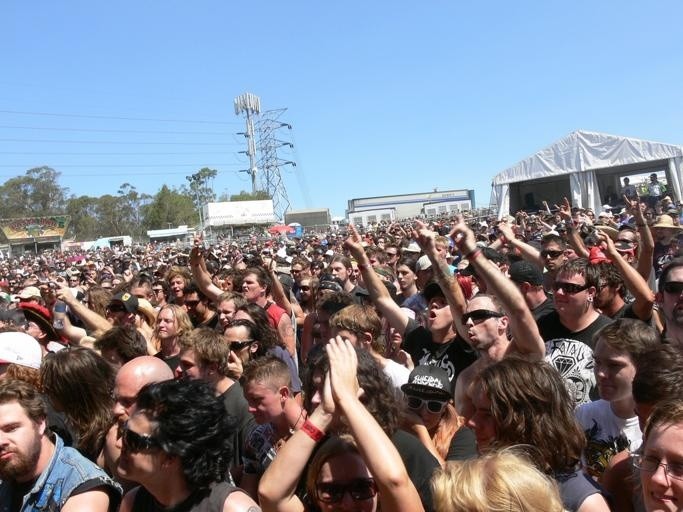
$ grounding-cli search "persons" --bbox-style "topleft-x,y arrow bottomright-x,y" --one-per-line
301,282 -> 341,368
94,323 -> 147,374
330,255 -> 364,305
310,347 -> 442,512
384,243 -> 400,275
496,198 -> 539,258
573,318 -> 644,486
1,237 -> 192,325
467,358 -> 611,512
194,231 -> 300,314
431,443 -> 564,512
642,401 -> 683,512
400,365 -> 478,469
305,295 -> 360,411
395,259 -> 423,303
435,258 -> 547,454
417,279 -> 477,399
40,348 -> 117,453
434,234 -> 458,280
540,196 -> 597,229
349,259 -> 364,288
224,320 -> 268,381
299,230 -> 328,282
539,234 -> 567,291
648,173 -> 666,200
602,344 -> 683,512
176,327 -> 258,470
650,255 -> 683,346
592,230 -> 654,323
365,247 -> 387,266
329,225 -> 349,255
349,206 -> 495,259
0,333 -> 43,377
151,305 -> 194,374
598,202 -> 683,231
395,257 -> 425,307
509,261 -> 558,343
0,380 -> 124,512
116,372 -> 263,512
385,307 -> 414,361
238,353 -> 309,504
605,175 -> 640,203
257,335 -> 425,512
96,355 -> 176,492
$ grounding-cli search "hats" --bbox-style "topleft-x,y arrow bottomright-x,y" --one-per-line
400,365 -> 452,401
507,260 -> 544,286
602,205 -> 612,210
19,302 -> 57,338
15,286 -> 41,299
111,291 -> 138,314
403,244 -> 421,253
652,214 -> 678,229
0,332 -> 42,370
137,298 -> 155,326
415,254 -> 432,272
422,283 -> 446,300
318,274 -> 344,292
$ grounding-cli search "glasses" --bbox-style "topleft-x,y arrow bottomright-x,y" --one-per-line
453,269 -> 463,273
405,395 -> 447,415
290,268 -> 304,273
387,253 -> 396,256
183,299 -> 200,306
312,477 -> 377,504
69,279 -> 77,282
461,309 -> 500,326
632,451 -> 683,482
616,239 -> 633,244
228,339 -> 253,350
8,282 -> 15,285
298,286 -> 310,291
115,426 -> 148,454
552,280 -> 587,293
541,250 -> 563,259
663,281 -> 683,295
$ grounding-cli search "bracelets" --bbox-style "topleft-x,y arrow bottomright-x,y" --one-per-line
300,418 -> 325,443
467,248 -> 482,263
